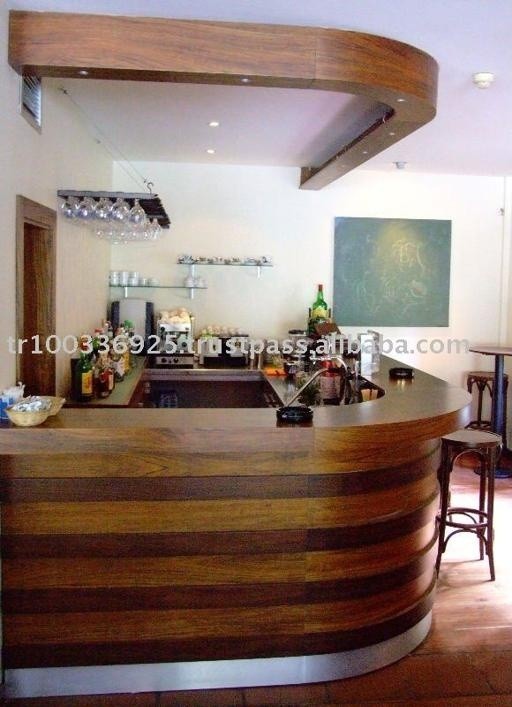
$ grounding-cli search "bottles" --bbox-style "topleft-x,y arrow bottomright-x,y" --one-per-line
312,284 -> 328,323
68,321 -> 136,402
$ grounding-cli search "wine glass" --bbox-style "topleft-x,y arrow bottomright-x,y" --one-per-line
62,190 -> 163,242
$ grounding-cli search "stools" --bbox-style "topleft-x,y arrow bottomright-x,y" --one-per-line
437,429 -> 502,580
467,371 -> 508,467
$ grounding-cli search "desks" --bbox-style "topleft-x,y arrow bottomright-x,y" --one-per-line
470,344 -> 512,478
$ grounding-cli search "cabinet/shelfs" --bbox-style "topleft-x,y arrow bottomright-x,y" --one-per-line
128,369 -> 149,408
143,370 -> 264,408
